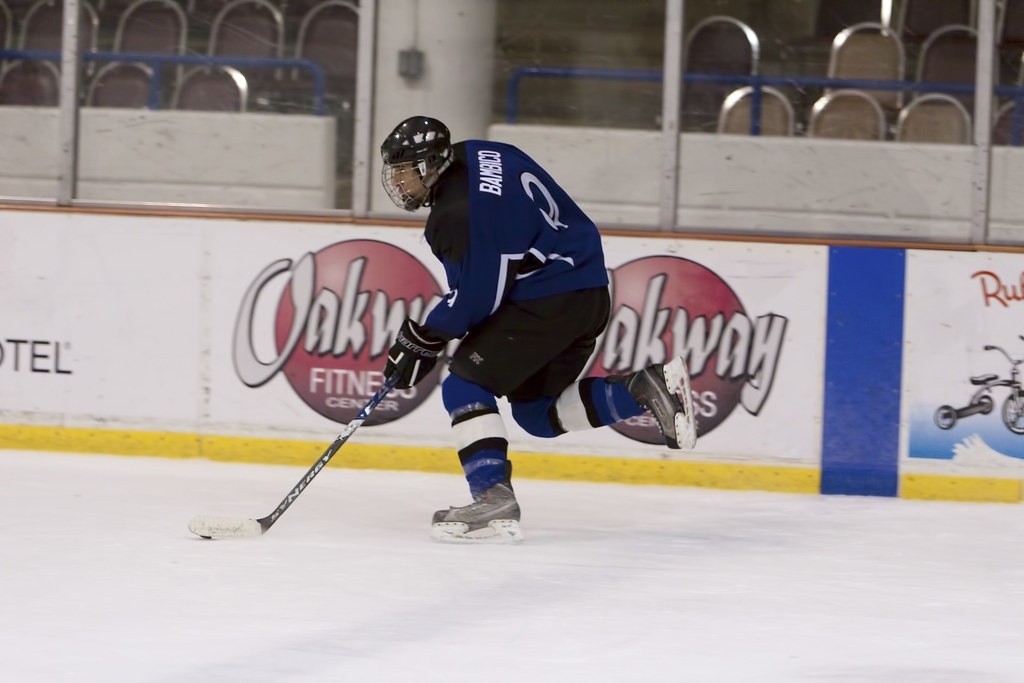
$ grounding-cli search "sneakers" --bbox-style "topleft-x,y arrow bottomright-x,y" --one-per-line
604,357 -> 696,450
432,460 -> 521,543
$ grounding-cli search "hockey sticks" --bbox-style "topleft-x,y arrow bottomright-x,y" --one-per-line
188,372 -> 399,538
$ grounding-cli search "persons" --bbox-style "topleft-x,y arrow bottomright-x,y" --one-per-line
380,115 -> 697,546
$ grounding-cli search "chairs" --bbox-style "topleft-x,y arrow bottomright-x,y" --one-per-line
0,0 -> 360,112
682,0 -> 1023,149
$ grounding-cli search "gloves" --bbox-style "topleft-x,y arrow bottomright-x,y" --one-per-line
383,315 -> 447,389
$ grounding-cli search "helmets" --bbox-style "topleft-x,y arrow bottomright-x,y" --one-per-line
381,116 -> 452,212
